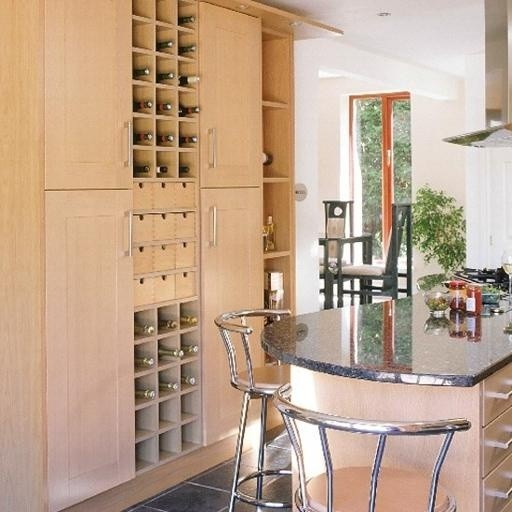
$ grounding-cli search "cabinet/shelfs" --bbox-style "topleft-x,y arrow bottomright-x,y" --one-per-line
287,360 -> 512,512
2,1 -> 344,512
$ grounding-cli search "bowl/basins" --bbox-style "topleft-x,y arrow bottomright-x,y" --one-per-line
423,312 -> 454,337
424,289 -> 454,314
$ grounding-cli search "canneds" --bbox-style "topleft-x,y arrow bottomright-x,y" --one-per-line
463,314 -> 482,341
447,281 -> 466,310
448,310 -> 465,337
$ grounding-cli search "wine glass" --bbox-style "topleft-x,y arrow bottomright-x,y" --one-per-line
502,247 -> 512,301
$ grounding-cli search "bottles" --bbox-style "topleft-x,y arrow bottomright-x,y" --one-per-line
180,166 -> 190,174
463,311 -> 483,345
180,313 -> 198,328
158,319 -> 179,332
180,45 -> 197,55
178,103 -> 201,117
178,74 -> 200,87
178,15 -> 197,26
264,215 -> 276,252
181,344 -> 199,355
179,135 -> 198,148
132,68 -> 150,81
155,41 -> 173,53
157,165 -> 168,175
155,72 -> 173,84
158,347 -> 184,360
156,134 -> 174,145
134,357 -> 154,369
134,322 -> 155,337
134,164 -> 150,177
159,382 -> 179,391
181,375 -> 196,386
134,131 -> 153,145
134,100 -> 153,113
157,102 -> 172,116
135,388 -> 156,400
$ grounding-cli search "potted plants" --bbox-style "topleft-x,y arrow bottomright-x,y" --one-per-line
408,182 -> 469,295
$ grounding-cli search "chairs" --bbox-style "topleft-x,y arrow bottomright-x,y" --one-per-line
336,200 -> 412,309
214,306 -> 295,512
318,200 -> 357,311
271,379 -> 474,512
